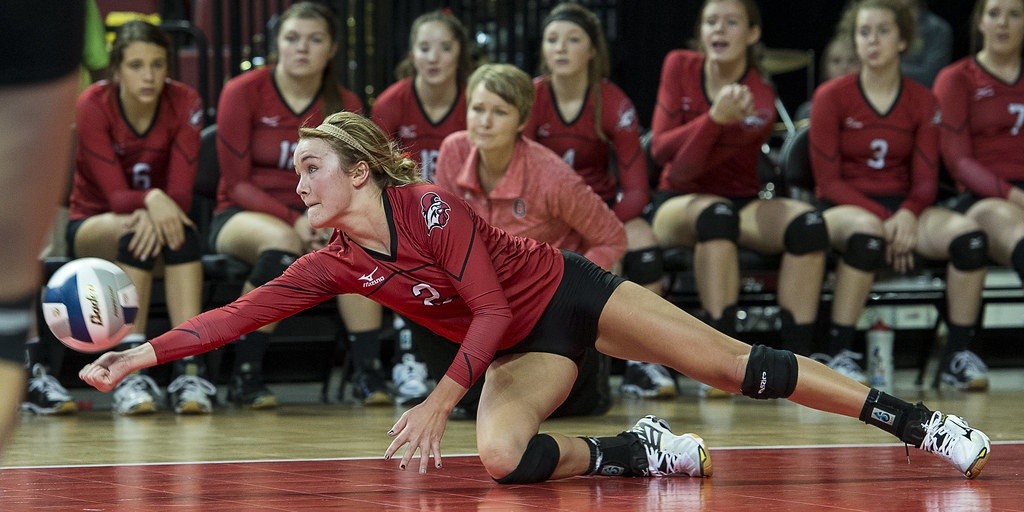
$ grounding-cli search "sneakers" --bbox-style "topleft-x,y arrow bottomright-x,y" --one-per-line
899,401 -> 993,481
826,349 -> 870,387
167,360 -> 219,415
939,349 -> 989,392
223,360 -> 278,409
616,415 -> 713,480
19,362 -> 78,414
389,353 -> 431,406
619,360 -> 676,399
111,372 -> 163,415
697,382 -> 736,398
350,369 -> 392,406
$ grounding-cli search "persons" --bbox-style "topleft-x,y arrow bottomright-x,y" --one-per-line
513,0 -> 678,399
801,1 -> 1024,387
78,112 -> 991,487
207,0 -> 391,411
63,19 -> 216,415
21,324 -> 78,417
433,63 -> 626,420
0,0 -> 90,462
654,0 -> 832,399
368,10 -> 472,405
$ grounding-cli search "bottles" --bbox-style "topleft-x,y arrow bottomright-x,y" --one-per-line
867,321 -> 894,396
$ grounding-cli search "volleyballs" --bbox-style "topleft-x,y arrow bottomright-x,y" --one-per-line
41,257 -> 139,353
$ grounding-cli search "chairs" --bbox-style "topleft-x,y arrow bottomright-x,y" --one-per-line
187,127 -> 1024,403
